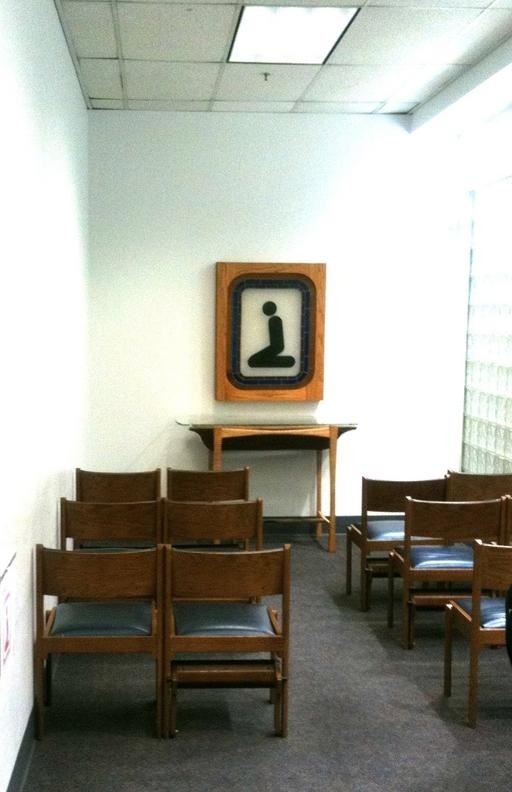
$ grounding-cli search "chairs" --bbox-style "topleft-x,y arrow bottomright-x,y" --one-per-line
163,542 -> 289,739
35,543 -> 164,737
57,466 -> 266,605
389,494 -> 512,651
441,535 -> 510,727
345,468 -> 511,609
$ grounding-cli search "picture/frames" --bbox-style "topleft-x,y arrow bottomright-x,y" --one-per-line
183,421 -> 358,553
215,261 -> 324,404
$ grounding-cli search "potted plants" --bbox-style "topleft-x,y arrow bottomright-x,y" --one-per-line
215,261 -> 324,404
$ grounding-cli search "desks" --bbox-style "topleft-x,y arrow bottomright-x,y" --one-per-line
183,421 -> 358,553
